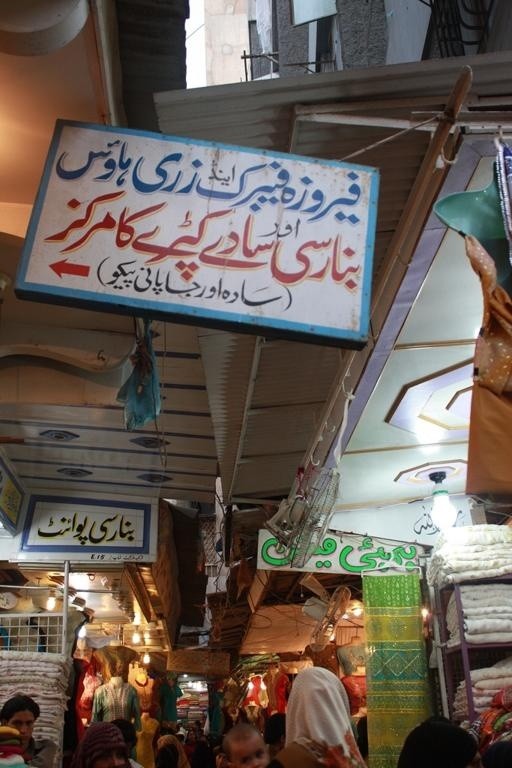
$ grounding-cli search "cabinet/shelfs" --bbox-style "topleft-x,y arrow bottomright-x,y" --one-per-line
426,556 -> 512,767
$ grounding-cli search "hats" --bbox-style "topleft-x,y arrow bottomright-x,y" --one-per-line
163,670 -> 178,679
127,668 -> 155,696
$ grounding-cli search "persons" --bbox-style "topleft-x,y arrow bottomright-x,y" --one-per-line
267,664 -> 366,767
1,694 -> 284,768
396,715 -> 481,768
483,741 -> 511,767
263,662 -> 291,718
283,667 -> 299,701
0,599 -> 160,768
335,637 -> 366,675
304,635 -> 340,675
432,154 -> 511,530
160,678 -> 184,732
341,667 -> 366,718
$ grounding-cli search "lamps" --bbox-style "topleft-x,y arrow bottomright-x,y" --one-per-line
45,589 -> 151,665
432,477 -> 457,532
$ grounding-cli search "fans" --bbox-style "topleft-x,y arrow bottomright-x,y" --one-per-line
223,679 -> 251,710
299,572 -> 352,653
264,466 -> 341,569
